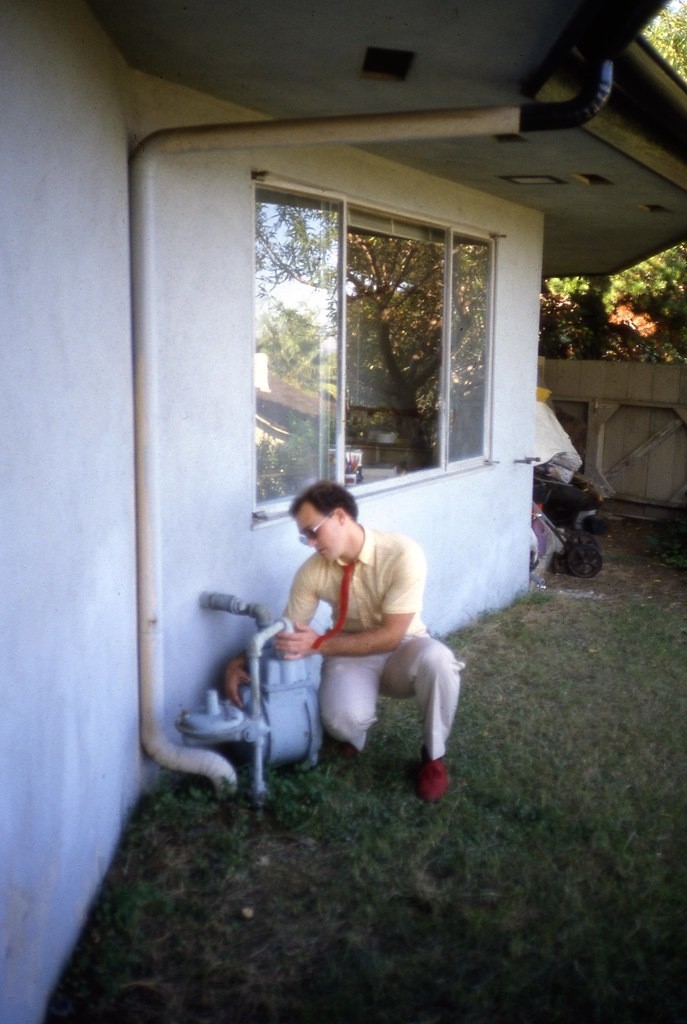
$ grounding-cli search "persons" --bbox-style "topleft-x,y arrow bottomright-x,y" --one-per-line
224,479 -> 462,803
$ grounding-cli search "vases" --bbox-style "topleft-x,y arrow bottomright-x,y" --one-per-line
375,433 -> 399,445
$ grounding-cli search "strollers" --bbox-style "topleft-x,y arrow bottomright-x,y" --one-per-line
532,464 -> 607,578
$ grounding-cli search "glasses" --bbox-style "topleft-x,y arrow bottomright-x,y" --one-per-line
299,513 -> 335,545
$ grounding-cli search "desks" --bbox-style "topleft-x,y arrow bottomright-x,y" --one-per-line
347,441 -> 436,463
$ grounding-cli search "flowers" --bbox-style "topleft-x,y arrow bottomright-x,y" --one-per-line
368,408 -> 400,434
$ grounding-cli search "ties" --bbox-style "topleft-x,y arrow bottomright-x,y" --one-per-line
312,564 -> 355,649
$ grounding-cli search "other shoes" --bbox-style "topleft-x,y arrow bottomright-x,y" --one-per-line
338,741 -> 355,758
416,747 -> 448,804
